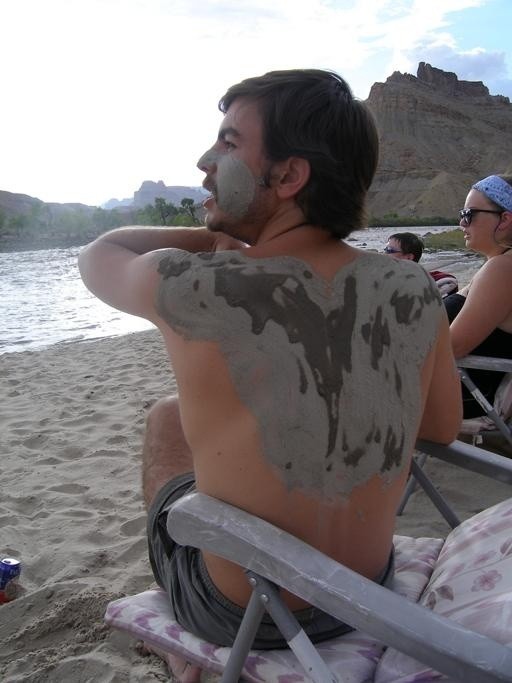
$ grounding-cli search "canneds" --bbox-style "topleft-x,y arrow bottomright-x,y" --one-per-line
0,557 -> 21,603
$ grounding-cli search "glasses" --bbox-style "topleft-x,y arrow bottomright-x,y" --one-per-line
383,246 -> 404,252
459,208 -> 500,223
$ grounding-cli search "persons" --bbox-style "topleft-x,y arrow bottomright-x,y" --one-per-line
382,232 -> 425,264
443,175 -> 512,419
78,69 -> 463,649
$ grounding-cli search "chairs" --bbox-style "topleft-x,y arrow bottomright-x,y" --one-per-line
104,439 -> 511,682
395,354 -> 511,530
